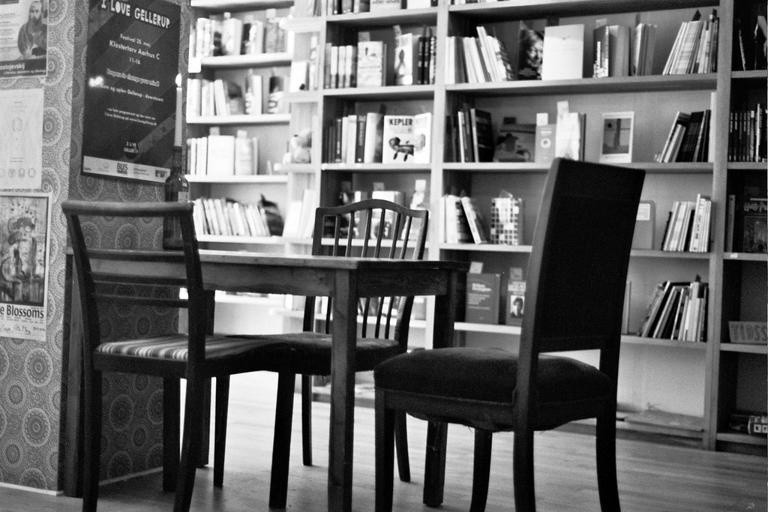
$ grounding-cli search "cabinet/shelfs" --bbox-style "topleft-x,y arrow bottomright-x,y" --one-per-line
188,0 -> 768,450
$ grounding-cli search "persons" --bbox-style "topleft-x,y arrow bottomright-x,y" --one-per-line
18,2 -> 46,71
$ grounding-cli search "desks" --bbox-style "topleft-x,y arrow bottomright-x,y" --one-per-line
186,246 -> 472,511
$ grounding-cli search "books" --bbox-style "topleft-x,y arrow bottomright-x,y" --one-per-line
311,293 -> 431,322
442,99 -> 712,252
639,272 -> 709,343
719,194 -> 767,345
463,259 -> 527,325
324,102 -> 433,240
184,13 -> 295,115
726,3 -> 768,163
310,0 -> 440,88
185,125 -> 281,239
441,0 -> 719,80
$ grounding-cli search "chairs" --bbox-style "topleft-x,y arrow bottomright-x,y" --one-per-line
59,200 -> 299,512
302,197 -> 434,483
373,153 -> 647,512
